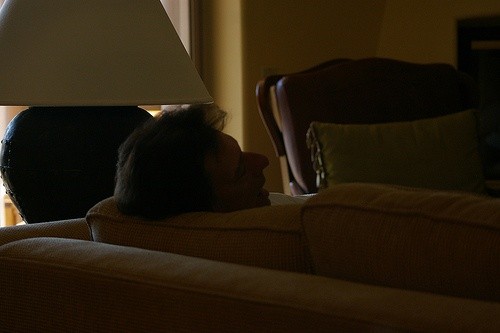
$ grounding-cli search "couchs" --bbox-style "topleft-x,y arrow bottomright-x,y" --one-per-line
0,190 -> 500,333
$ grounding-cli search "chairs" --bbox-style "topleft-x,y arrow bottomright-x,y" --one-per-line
254,57 -> 500,197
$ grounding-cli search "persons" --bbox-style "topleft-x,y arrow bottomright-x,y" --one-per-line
110,103 -> 274,217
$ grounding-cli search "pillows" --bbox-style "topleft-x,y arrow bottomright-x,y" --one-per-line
305,107 -> 488,196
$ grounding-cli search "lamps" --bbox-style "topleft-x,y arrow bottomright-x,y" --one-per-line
0,0 -> 215,220
